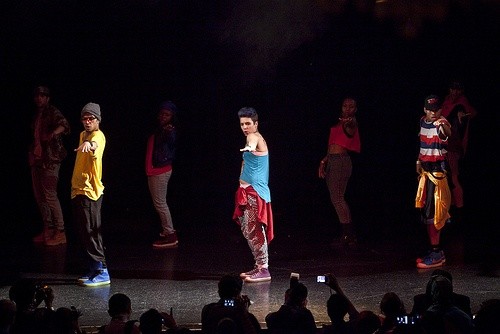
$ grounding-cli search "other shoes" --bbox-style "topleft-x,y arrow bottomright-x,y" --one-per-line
417,253 -> 442,268
34,230 -> 56,242
152,232 -> 178,247
416,250 -> 447,262
334,232 -> 357,244
46,232 -> 67,246
77,261 -> 111,287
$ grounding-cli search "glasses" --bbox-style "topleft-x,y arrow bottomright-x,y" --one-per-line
81,117 -> 96,121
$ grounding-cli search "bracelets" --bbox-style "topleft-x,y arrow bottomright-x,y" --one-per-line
416,161 -> 421,165
82,139 -> 92,147
321,160 -> 325,164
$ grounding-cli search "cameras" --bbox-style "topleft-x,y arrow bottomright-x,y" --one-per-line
316,276 -> 326,283
397,316 -> 408,324
223,300 -> 235,307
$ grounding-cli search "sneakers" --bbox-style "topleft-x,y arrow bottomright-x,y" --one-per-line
240,268 -> 262,278
246,269 -> 272,282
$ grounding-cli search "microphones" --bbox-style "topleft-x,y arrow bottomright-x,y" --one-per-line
462,113 -> 471,117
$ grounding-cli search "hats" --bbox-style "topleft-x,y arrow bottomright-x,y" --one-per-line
425,95 -> 440,111
34,86 -> 49,96
430,274 -> 453,299
160,100 -> 177,113
81,102 -> 102,123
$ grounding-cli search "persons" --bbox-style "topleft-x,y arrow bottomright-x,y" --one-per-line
201,271 -> 500,334
145,101 -> 178,247
0,278 -> 88,334
71,102 -> 111,286
415,94 -> 452,269
98,294 -> 194,334
441,79 -> 476,208
232,106 -> 274,283
28,86 -> 70,246
318,97 -> 361,247
